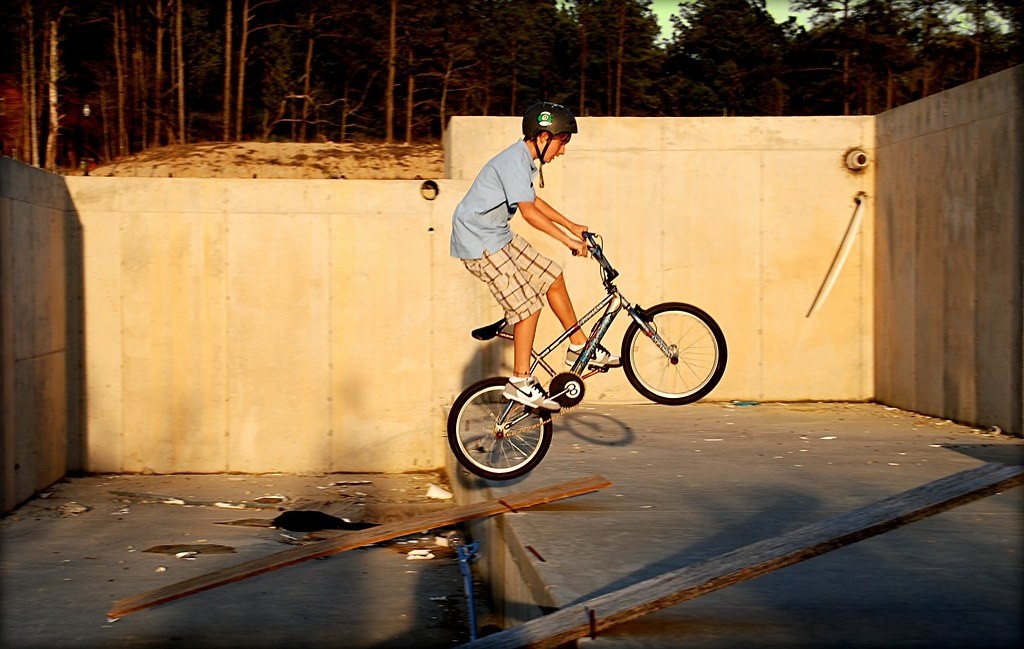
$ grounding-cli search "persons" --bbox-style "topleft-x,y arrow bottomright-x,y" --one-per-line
448,102 -> 623,413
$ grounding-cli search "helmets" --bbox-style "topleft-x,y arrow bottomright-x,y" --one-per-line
522,102 -> 579,133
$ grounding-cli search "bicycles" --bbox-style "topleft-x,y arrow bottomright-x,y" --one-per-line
446,231 -> 728,483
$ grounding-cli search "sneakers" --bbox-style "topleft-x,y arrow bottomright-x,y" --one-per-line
503,377 -> 560,412
565,347 -> 622,368
